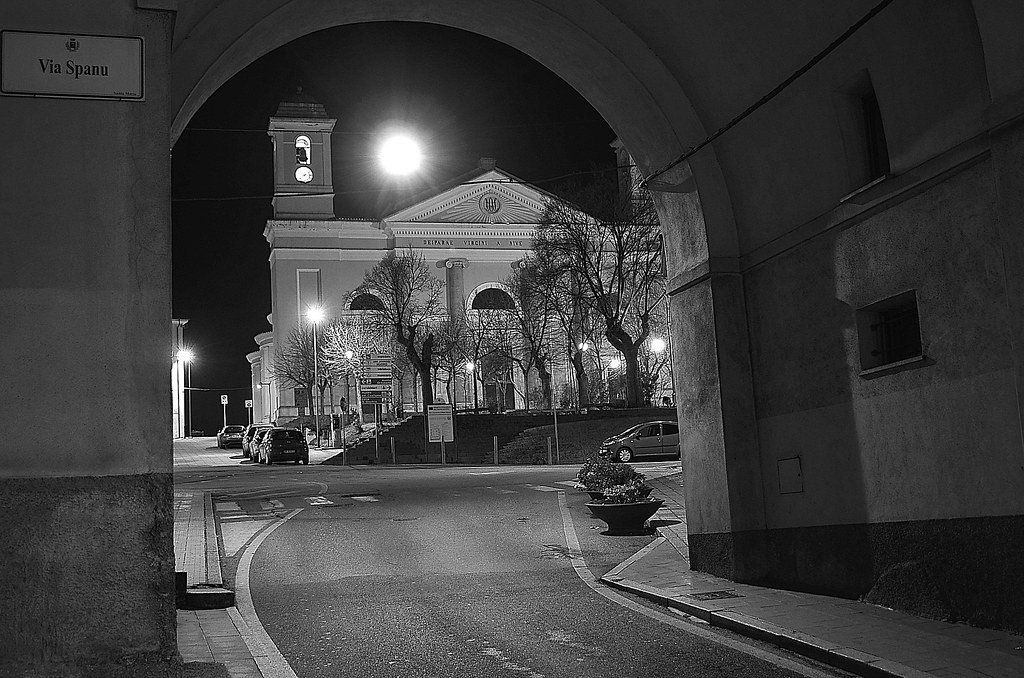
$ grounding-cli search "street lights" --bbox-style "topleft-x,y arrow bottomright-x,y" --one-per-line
307,305 -> 326,446
177,350 -> 196,437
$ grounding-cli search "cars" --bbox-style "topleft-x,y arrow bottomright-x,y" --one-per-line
241,421 -> 274,457
248,427 -> 273,461
217,424 -> 247,449
258,427 -> 310,465
599,420 -> 680,463
